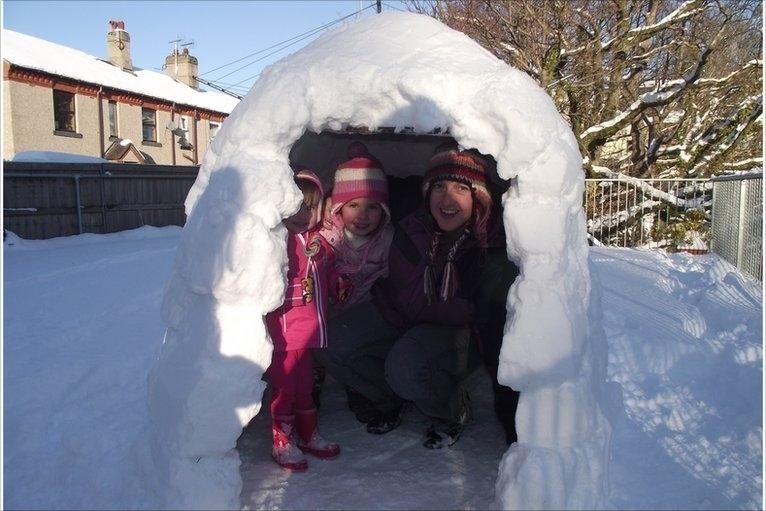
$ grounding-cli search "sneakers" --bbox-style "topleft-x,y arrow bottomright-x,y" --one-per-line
345,386 -> 472,450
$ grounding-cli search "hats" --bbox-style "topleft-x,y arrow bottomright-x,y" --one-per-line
420,141 -> 494,307
292,166 -> 324,225
331,141 -> 391,275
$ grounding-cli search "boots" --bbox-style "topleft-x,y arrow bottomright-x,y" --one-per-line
294,405 -> 339,461
270,416 -> 308,473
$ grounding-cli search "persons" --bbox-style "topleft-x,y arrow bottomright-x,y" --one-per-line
318,144 -> 494,451
314,155 -> 396,429
265,166 -> 343,474
